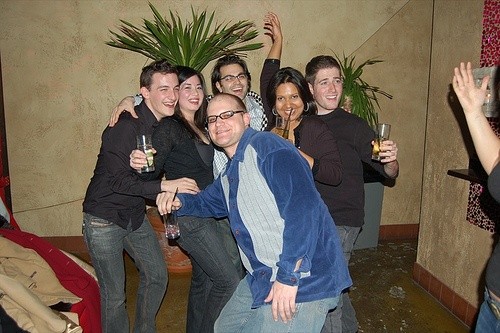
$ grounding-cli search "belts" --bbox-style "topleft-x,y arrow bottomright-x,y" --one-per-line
485,289 -> 500,318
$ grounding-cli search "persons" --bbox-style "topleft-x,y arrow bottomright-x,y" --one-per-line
81,11 -> 399,333
452,61 -> 500,333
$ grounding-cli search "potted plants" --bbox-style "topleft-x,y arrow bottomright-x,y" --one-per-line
104,0 -> 264,273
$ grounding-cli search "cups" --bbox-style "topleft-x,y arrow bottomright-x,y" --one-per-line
276,116 -> 289,141
167,210 -> 180,246
136,135 -> 155,174
372,123 -> 391,161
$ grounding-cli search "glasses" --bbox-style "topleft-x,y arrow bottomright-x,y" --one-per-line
218,73 -> 247,82
205,111 -> 244,123
